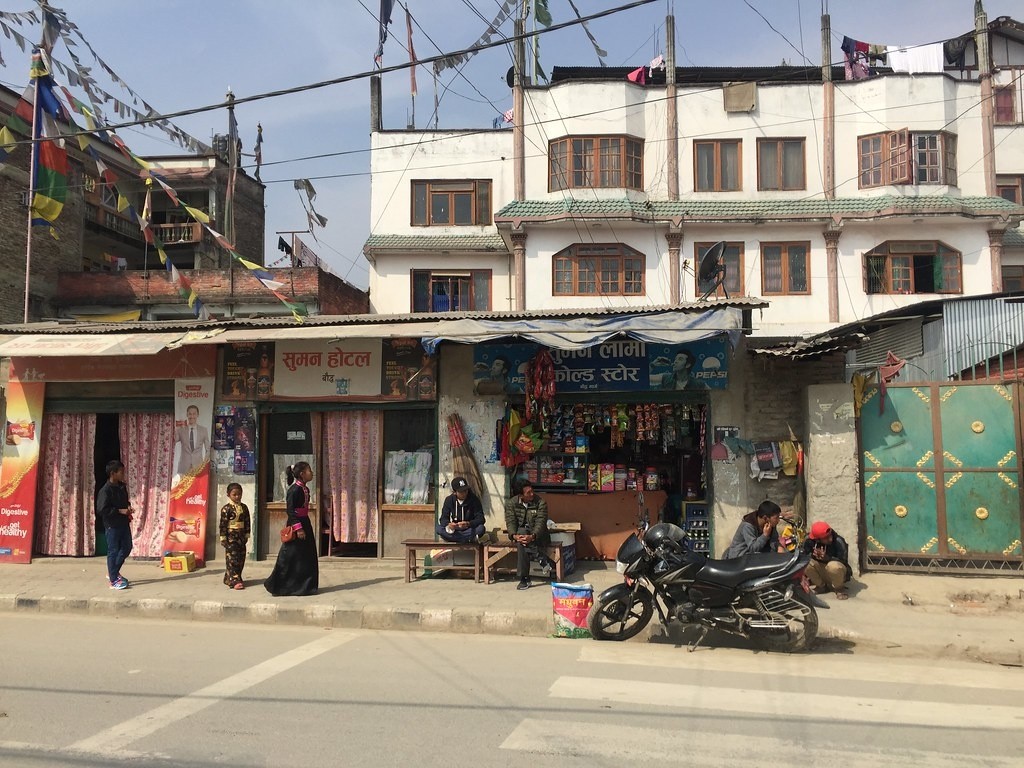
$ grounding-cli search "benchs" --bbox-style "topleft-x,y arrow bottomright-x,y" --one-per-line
401,537 -> 482,583
483,540 -> 565,583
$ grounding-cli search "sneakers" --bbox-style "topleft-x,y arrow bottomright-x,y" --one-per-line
106,573 -> 126,582
110,579 -> 127,589
517,579 -> 532,589
539,559 -> 552,575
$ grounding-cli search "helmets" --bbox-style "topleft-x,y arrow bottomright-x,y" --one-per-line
645,523 -> 690,552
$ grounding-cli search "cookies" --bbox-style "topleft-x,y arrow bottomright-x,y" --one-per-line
168,531 -> 187,543
6,434 -> 21,445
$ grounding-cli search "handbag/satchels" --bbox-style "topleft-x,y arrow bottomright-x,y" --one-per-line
280,526 -> 295,542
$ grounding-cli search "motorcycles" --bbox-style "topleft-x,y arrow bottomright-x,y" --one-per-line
586,491 -> 831,653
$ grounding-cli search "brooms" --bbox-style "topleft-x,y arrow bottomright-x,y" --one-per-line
445,413 -> 484,502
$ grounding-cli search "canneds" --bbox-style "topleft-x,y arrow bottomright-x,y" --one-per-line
241,456 -> 247,471
406,367 -> 418,400
245,368 -> 257,400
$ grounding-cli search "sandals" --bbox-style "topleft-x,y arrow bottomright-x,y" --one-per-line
810,584 -> 830,593
834,587 -> 848,599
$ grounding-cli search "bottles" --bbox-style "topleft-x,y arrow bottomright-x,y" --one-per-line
643,468 -> 660,491
658,358 -> 675,367
419,351 -> 434,400
257,346 -> 270,397
687,530 -> 710,540
686,480 -> 697,501
615,464 -> 627,490
476,363 -> 492,371
689,521 -> 707,528
693,508 -> 703,516
628,468 -> 639,490
695,543 -> 705,550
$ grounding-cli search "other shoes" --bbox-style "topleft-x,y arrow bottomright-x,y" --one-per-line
469,534 -> 480,544
233,583 -> 243,590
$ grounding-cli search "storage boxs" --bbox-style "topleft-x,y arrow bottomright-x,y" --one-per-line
679,498 -> 710,561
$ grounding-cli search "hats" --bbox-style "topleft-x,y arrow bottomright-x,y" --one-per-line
809,522 -> 831,539
451,477 -> 470,491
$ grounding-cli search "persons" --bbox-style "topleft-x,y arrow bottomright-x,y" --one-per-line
474,356 -> 525,393
97,460 -> 135,589
649,348 -> 711,390
219,483 -> 251,589
722,501 -> 781,560
176,405 -> 210,480
506,479 -> 553,590
438,477 -> 486,542
804,522 -> 853,600
264,462 -> 319,596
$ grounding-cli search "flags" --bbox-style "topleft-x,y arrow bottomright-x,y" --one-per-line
0,49 -> 299,318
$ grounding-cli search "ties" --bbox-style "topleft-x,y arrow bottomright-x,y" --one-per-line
191,427 -> 195,452
